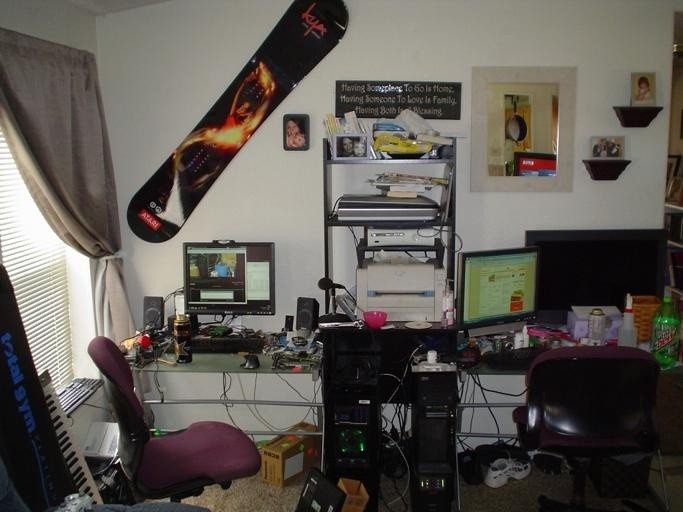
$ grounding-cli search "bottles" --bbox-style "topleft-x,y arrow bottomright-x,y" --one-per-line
650,285 -> 680,370
172,313 -> 191,364
587,307 -> 605,345
494,326 -> 529,351
618,293 -> 637,348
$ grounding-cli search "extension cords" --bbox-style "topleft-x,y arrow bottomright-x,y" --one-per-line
380,435 -> 401,448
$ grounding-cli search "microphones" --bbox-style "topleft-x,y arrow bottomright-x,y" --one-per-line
318,277 -> 345,289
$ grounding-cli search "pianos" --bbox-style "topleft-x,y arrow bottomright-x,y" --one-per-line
37,368 -> 104,505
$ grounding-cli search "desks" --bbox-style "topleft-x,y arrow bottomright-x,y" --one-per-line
455,354 -> 682,457
130,350 -> 327,473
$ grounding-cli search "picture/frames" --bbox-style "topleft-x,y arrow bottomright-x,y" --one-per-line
282,113 -> 310,151
627,70 -> 658,108
331,133 -> 370,159
589,135 -> 626,161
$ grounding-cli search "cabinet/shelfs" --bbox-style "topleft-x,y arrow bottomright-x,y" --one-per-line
321,136 -> 457,323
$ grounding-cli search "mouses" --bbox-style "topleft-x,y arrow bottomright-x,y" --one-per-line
243,355 -> 260,369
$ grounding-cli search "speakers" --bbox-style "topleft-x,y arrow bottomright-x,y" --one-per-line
296,297 -> 319,330
143,296 -> 165,330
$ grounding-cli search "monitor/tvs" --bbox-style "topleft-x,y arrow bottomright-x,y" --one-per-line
183,241 -> 275,315
525,228 -> 668,323
456,246 -> 541,338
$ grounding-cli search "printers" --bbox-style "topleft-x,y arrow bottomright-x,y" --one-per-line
356,258 -> 445,323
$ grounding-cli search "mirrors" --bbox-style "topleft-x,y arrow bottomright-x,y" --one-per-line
468,66 -> 576,194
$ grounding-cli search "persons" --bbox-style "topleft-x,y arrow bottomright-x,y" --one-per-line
353,137 -> 367,157
286,120 -> 301,147
592,137 -> 620,156
341,137 -> 354,157
632,78 -> 655,107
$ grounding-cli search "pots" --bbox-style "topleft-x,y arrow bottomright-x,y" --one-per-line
502,95 -> 529,145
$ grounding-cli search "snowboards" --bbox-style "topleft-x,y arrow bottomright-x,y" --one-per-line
127,0 -> 349,243
1,266 -> 78,512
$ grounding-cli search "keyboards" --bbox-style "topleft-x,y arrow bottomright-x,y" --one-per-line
184,339 -> 264,353
485,347 -> 545,370
55,378 -> 104,416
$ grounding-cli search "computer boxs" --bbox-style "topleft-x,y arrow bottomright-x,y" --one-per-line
330,401 -> 381,512
411,371 -> 458,512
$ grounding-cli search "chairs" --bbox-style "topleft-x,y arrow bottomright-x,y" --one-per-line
511,346 -> 663,512
87,336 -> 260,503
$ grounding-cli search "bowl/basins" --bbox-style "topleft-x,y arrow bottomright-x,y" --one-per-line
363,310 -> 386,328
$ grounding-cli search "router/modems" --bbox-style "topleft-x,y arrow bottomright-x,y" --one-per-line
202,325 -> 233,336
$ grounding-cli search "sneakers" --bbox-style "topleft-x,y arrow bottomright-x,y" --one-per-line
483,459 -> 531,489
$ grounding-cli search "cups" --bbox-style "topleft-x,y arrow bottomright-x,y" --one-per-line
426,350 -> 436,363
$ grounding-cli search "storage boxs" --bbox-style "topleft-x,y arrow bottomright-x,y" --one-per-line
259,421 -> 318,489
563,299 -> 621,342
336,477 -> 369,512
